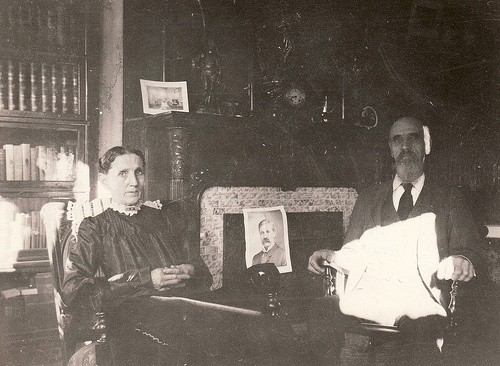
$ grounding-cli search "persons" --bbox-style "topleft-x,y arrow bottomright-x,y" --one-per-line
62,146 -> 217,366
308,117 -> 478,366
252,219 -> 288,267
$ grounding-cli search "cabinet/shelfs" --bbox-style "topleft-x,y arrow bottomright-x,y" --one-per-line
0,1 -> 106,366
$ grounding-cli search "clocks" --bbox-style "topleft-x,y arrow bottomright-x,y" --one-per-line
284,84 -> 306,109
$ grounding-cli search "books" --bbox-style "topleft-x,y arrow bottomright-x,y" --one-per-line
0,58 -> 78,182
16,211 -> 46,249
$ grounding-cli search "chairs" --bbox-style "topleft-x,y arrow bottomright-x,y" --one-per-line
313,253 -> 461,366
40,199 -> 283,366
